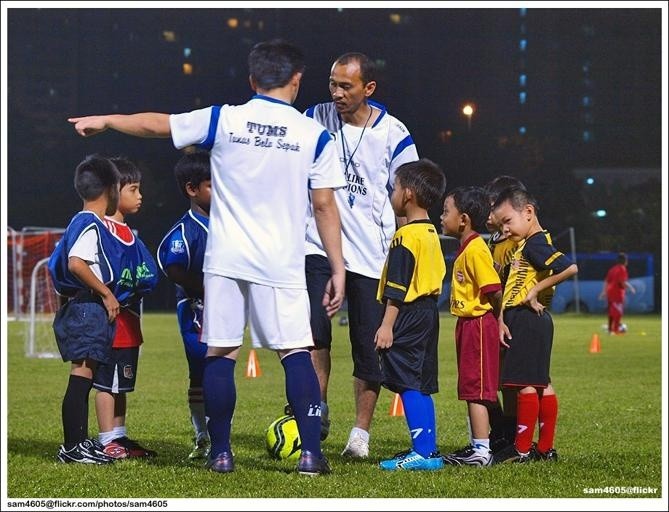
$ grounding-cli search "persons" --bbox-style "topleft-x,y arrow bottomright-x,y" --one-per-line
439,186 -> 503,468
491,186 -> 580,464
48,156 -> 122,465
597,252 -> 636,335
488,175 -> 527,453
66,39 -> 348,478
375,158 -> 446,470
155,153 -> 211,467
94,156 -> 158,462
302,52 -> 421,461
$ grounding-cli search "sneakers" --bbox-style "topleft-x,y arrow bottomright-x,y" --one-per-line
342,427 -> 369,459
285,401 -> 331,441
379,448 -> 443,471
58,437 -> 157,465
443,427 -> 557,467
188,437 -> 233,473
296,450 -> 330,476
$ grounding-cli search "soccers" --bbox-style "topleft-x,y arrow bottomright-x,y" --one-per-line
619,323 -> 628,333
266,415 -> 302,462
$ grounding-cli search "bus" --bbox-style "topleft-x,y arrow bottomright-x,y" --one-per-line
436,234 -> 490,310
551,251 -> 655,315
